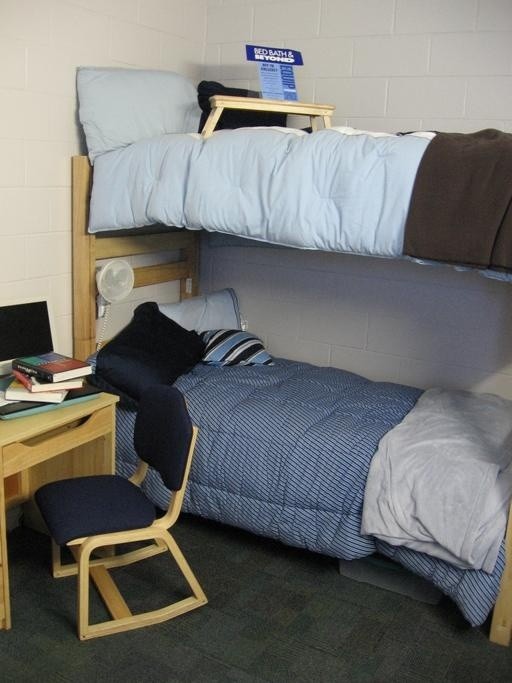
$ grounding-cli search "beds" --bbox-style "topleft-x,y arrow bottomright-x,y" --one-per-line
71,125 -> 511,648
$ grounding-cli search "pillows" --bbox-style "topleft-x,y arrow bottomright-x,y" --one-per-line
86,302 -> 277,411
74,65 -> 288,165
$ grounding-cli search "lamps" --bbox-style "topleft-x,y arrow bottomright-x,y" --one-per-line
91,258 -> 135,366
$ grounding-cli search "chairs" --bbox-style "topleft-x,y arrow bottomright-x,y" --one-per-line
33,384 -> 209,642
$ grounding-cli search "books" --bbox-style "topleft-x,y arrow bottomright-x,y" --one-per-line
5,375 -> 71,403
12,367 -> 85,392
12,350 -> 93,383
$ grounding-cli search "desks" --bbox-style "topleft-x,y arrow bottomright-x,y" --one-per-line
200,94 -> 336,141
0,391 -> 121,631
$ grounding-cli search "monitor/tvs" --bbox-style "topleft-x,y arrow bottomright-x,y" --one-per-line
0,301 -> 54,362
